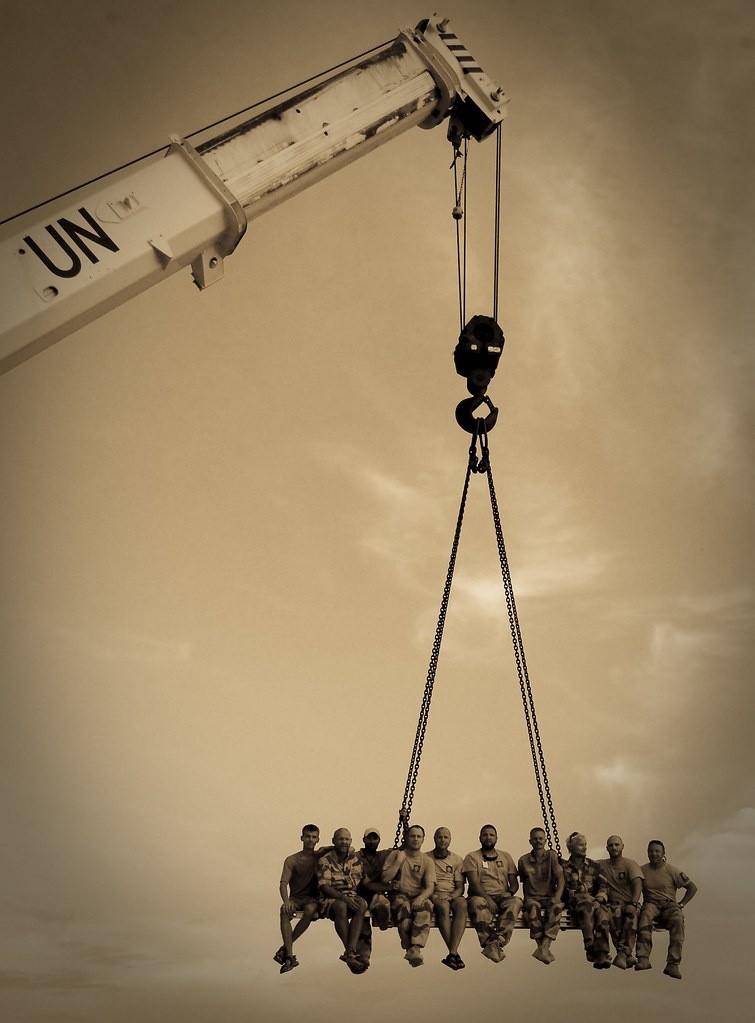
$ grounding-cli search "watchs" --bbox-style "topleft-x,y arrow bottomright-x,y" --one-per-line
678,902 -> 685,908
631,901 -> 638,911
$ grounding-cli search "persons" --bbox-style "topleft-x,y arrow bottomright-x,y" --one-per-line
462,824 -> 521,963
423,827 -> 468,970
381,825 -> 436,966
317,828 -> 372,973
633,840 -> 697,980
556,831 -> 612,970
273,824 -> 355,973
589,835 -> 644,970
353,806 -> 409,932
517,826 -> 567,966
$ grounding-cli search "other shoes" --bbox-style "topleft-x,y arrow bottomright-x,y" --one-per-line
403,948 -> 426,967
339,952 -> 370,974
481,945 -> 506,963
635,956 -> 651,971
370,903 -> 388,932
531,946 -> 556,965
663,963 -> 683,979
593,953 -> 612,969
613,951 -> 636,970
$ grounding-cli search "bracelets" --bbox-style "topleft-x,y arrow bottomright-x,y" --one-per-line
594,898 -> 603,905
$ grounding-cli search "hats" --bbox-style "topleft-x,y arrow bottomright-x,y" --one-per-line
364,828 -> 381,839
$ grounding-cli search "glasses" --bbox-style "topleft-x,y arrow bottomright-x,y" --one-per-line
570,832 -> 578,844
482,850 -> 498,861
433,850 -> 449,860
649,841 -> 663,846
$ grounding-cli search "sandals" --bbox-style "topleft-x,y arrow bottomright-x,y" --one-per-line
273,946 -> 299,973
442,954 -> 464,970
